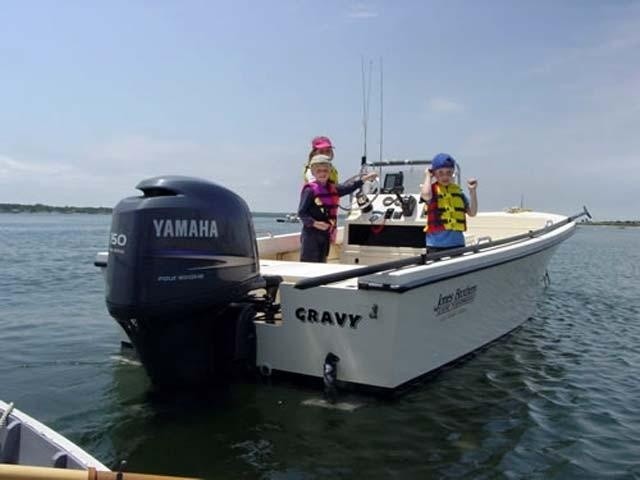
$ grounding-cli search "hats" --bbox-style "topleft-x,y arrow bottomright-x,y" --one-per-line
312,137 -> 331,149
432,153 -> 455,169
310,155 -> 330,166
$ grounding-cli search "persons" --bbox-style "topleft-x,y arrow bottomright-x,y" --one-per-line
296,154 -> 377,262
304,136 -> 338,184
419,153 -> 477,261
